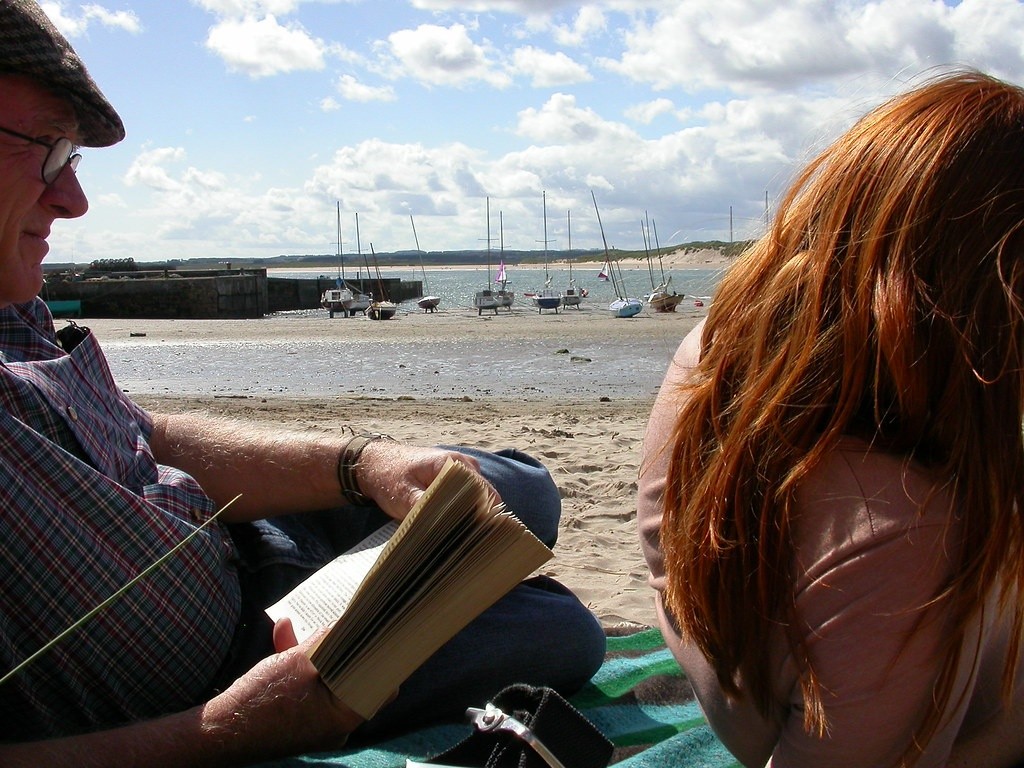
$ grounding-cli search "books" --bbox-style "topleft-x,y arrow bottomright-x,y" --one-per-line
263,458 -> 556,723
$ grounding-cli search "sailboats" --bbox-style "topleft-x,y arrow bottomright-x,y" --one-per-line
597,257 -> 611,283
410,214 -> 442,309
638,209 -> 686,310
319,201 -> 397,321
495,262 -> 514,285
532,190 -> 563,309
474,196 -> 518,310
561,209 -> 585,307
590,189 -> 644,318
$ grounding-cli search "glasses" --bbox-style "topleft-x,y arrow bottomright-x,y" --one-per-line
0,126 -> 82,184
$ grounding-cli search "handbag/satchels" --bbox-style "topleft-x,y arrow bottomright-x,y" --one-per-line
424,683 -> 616,768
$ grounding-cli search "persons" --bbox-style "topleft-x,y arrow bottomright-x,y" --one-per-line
0,0 -> 605,768
637,73 -> 1024,768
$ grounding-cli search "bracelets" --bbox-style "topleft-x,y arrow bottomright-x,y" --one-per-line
340,431 -> 401,505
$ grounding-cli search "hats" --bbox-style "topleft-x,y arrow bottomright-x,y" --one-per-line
0,0 -> 126,147
